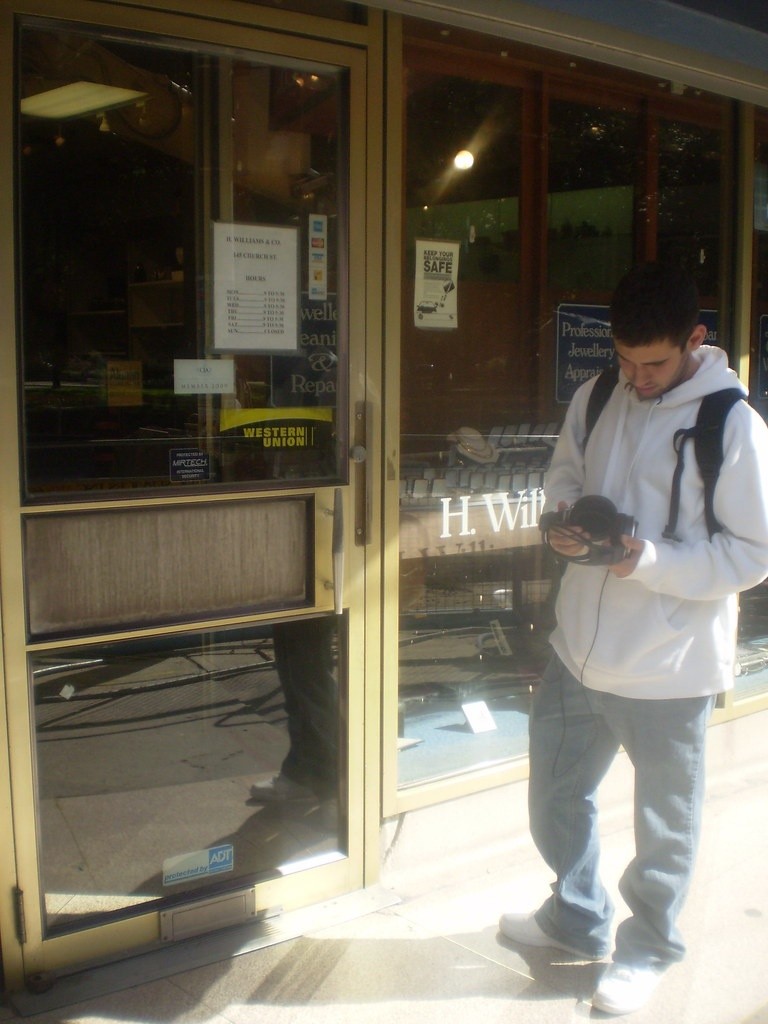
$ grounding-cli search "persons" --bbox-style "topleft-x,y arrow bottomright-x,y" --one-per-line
248,616 -> 338,804
498,260 -> 768,1016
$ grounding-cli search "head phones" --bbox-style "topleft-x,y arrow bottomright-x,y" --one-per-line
547,495 -> 636,566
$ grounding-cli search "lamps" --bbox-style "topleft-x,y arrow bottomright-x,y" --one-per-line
287,167 -> 319,184
167,71 -> 194,96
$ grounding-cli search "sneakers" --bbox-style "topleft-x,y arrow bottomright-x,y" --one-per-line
249,771 -> 314,803
593,951 -> 667,1016
499,912 -> 601,961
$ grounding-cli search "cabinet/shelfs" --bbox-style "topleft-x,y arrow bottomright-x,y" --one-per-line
64,212 -> 197,385
